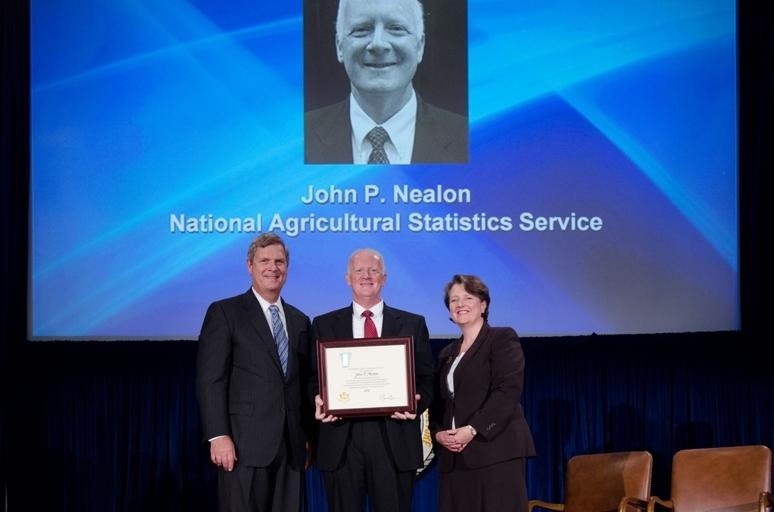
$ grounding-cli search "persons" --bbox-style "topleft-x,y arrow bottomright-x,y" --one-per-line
301,0 -> 471,165
190,231 -> 313,508
425,272 -> 538,511
307,248 -> 439,511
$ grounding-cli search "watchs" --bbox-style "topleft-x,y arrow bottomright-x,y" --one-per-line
467,423 -> 478,437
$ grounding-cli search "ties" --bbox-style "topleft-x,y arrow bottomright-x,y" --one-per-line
268,305 -> 289,377
362,310 -> 377,339
366,126 -> 390,164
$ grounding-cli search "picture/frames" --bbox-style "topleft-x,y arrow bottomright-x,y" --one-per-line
315,336 -> 416,418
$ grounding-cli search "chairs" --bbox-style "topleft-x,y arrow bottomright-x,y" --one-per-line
527,444 -> 773,512
